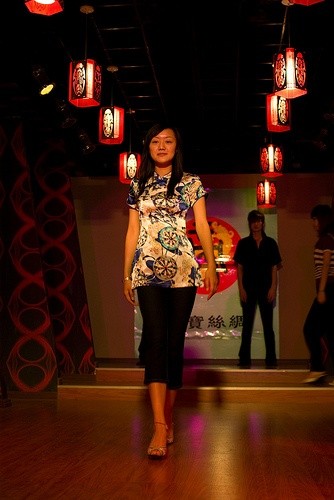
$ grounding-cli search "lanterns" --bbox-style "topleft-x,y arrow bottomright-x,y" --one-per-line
26,0 -> 64,17
118,152 -> 141,184
282,0 -> 324,7
256,47 -> 308,209
99,107 -> 125,145
69,58 -> 102,108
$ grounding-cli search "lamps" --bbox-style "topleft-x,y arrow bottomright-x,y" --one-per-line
68,5 -> 102,107
254,0 -> 326,210
119,109 -> 142,185
23,0 -> 64,17
99,65 -> 124,145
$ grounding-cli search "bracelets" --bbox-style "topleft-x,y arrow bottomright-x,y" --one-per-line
121,276 -> 132,281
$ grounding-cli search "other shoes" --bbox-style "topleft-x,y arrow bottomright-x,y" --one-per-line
147,435 -> 167,456
301,371 -> 327,383
166,428 -> 173,443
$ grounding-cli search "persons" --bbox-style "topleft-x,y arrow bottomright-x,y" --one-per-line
234,210 -> 283,369
302,205 -> 334,383
122,124 -> 219,457
